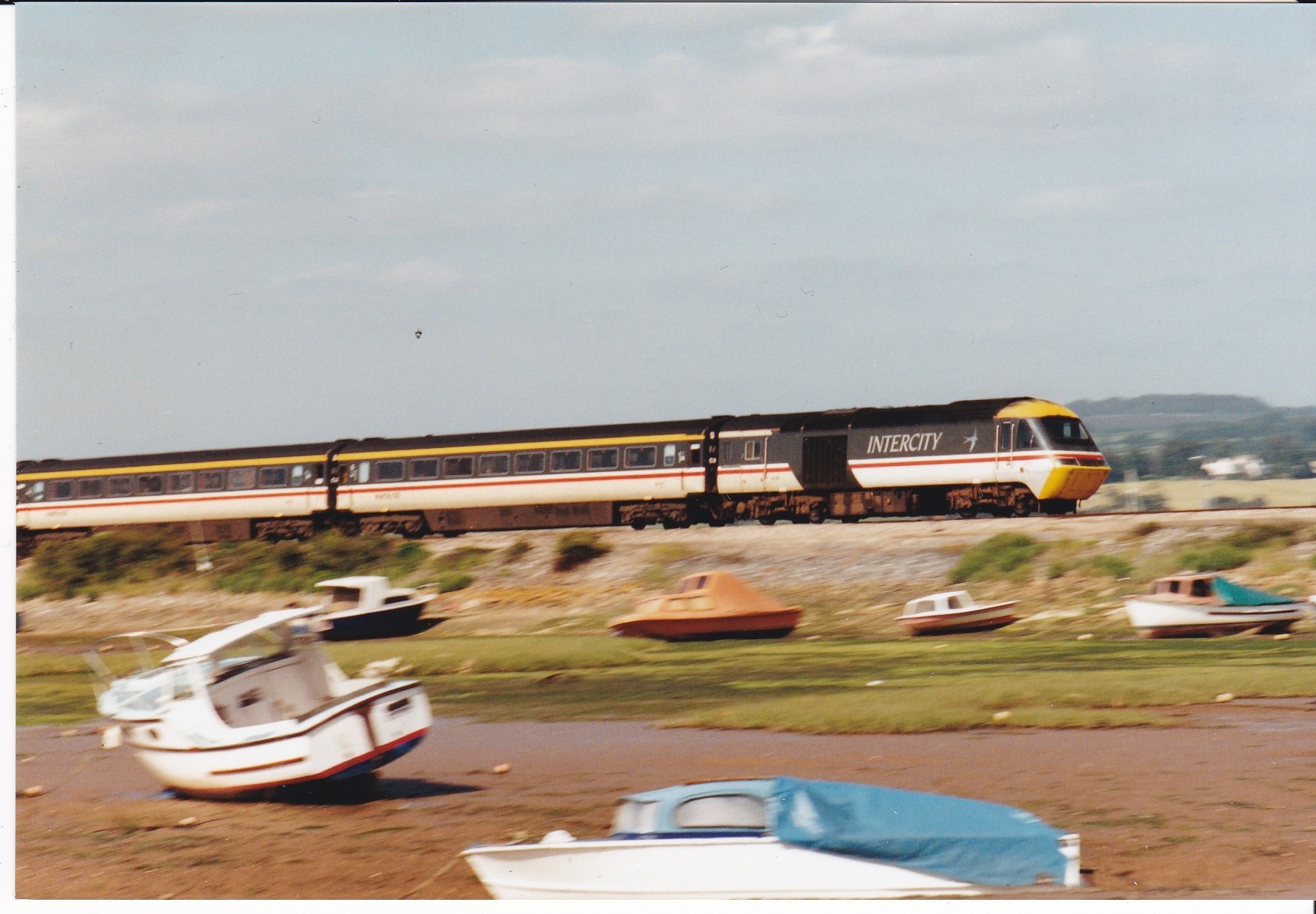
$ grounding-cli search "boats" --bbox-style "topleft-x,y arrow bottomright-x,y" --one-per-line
1120,569 -> 1310,640
258,576 -> 441,640
893,591 -> 1020,636
83,606 -> 431,797
607,572 -> 802,640
461,776 -> 1080,900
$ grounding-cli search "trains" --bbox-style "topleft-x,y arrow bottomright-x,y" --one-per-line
16,397 -> 1111,557
